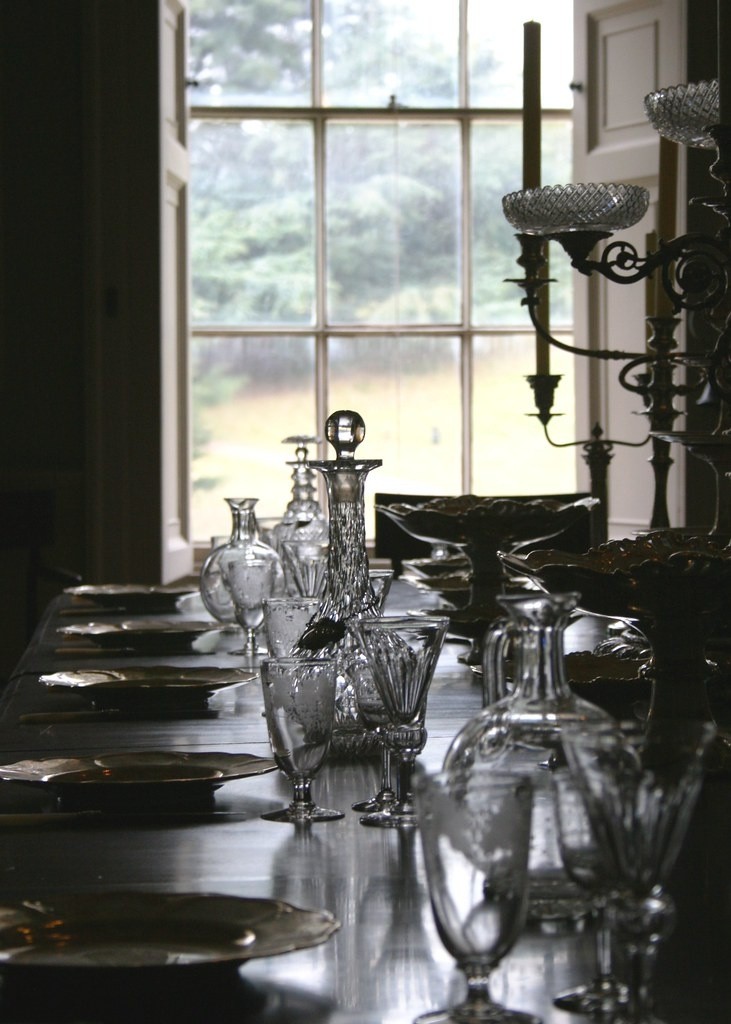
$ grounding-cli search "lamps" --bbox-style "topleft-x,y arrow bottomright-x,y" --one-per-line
507,0 -> 731,544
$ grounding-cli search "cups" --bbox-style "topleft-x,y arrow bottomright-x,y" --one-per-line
285,542 -> 394,618
262,596 -> 320,658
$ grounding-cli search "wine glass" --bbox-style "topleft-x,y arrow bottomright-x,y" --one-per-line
546,763 -> 635,1015
352,615 -> 452,830
217,558 -> 277,680
559,718 -> 717,1024
271,826 -> 345,919
412,767 -> 546,1024
261,657 -> 346,822
326,816 -> 448,1023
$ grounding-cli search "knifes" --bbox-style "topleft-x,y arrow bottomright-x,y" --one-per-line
0,811 -> 248,828
20,709 -> 220,724
61,608 -> 180,614
55,647 -> 214,654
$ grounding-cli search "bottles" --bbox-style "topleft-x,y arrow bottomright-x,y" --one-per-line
451,592 -> 643,902
284,409 -> 423,751
269,434 -> 331,599
199,497 -> 285,631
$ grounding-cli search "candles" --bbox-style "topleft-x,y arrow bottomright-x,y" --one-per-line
536,240 -> 551,376
645,231 -> 659,370
521,20 -> 541,191
657,138 -> 677,315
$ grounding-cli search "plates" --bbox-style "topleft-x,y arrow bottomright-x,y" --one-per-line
0,751 -> 280,808
62,584 -> 196,611
401,554 -> 560,638
57,619 -> 230,653
1,887 -> 343,983
38,665 -> 257,701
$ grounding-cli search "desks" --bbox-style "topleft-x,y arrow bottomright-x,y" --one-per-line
0,572 -> 731,1023
